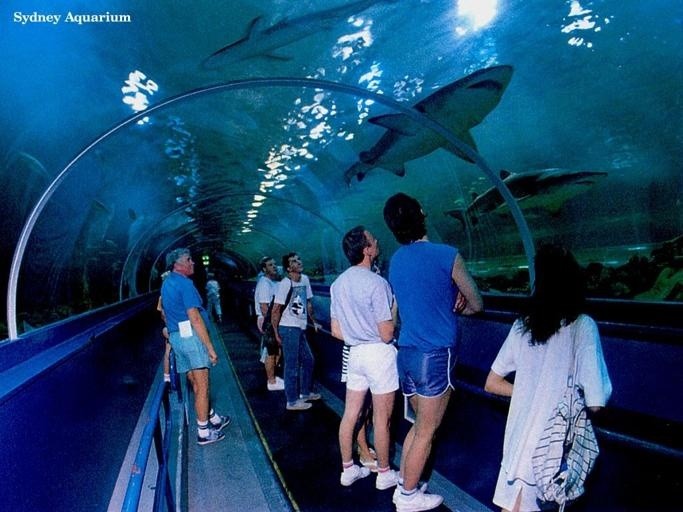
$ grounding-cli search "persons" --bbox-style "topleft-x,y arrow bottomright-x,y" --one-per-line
339,343 -> 379,475
269,249 -> 325,413
480,240 -> 616,511
253,255 -> 286,393
327,223 -> 400,492
382,191 -> 486,512
159,245 -> 232,449
155,270 -> 174,385
256,255 -> 284,358
203,273 -> 224,325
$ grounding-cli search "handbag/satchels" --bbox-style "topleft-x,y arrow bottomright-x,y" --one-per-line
263,323 -> 279,355
532,387 -> 599,511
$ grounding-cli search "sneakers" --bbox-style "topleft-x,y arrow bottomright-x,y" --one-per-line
267,376 -> 285,390
287,400 -> 313,410
341,448 -> 444,511
300,392 -> 320,401
197,415 -> 229,445
